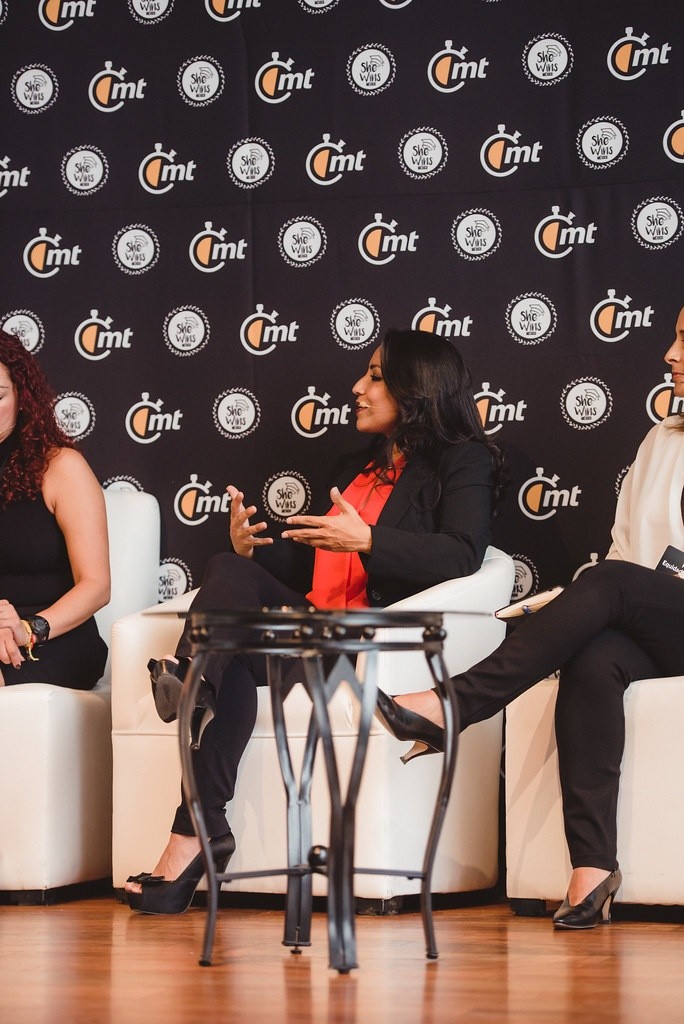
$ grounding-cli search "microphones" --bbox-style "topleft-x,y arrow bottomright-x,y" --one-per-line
360,401 -> 392,409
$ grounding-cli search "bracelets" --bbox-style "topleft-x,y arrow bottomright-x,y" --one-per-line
19,642 -> 19,647
20,620 -> 40,662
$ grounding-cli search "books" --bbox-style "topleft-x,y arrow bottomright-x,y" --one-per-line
495,585 -> 564,628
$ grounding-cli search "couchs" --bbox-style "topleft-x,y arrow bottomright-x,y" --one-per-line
503,670 -> 683,916
0,489 -> 162,907
111,541 -> 514,911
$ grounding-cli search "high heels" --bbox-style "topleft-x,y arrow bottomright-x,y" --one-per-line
551,868 -> 621,928
147,656 -> 217,749
124,831 -> 236,915
375,687 -> 446,765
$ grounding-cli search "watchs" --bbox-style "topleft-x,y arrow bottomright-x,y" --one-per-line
24,613 -> 50,647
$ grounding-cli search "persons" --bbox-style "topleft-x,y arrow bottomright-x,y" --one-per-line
0,330 -> 112,692
121,331 -> 510,917
376,310 -> 684,932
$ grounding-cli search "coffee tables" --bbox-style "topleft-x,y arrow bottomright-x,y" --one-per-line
146,605 -> 462,976
